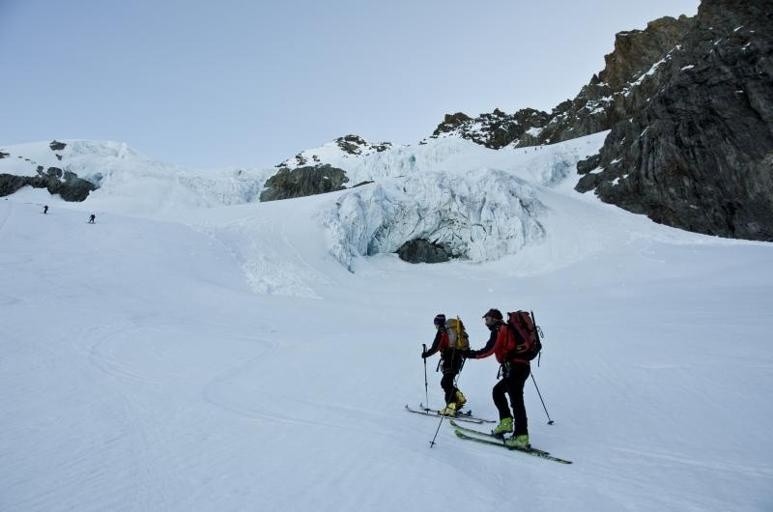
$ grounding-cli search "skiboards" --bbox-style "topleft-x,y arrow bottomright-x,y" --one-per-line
449,420 -> 573,466
404,403 -> 497,425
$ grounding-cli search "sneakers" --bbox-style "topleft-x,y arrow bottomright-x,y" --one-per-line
455,391 -> 465,411
505,435 -> 529,449
494,418 -> 513,433
438,403 -> 457,417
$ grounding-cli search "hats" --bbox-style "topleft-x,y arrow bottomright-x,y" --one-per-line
482,309 -> 502,320
434,315 -> 445,324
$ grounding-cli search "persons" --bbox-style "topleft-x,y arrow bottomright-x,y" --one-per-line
463,308 -> 532,449
420,313 -> 465,417
88,214 -> 95,224
44,205 -> 48,214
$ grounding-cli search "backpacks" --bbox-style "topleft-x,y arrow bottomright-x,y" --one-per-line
444,319 -> 469,350
507,312 -> 542,360
